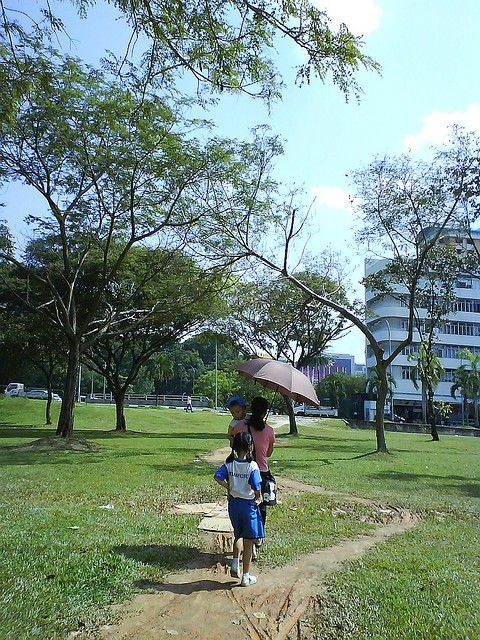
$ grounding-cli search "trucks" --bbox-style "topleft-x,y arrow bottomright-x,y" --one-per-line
4,383 -> 48,399
293,401 -> 335,417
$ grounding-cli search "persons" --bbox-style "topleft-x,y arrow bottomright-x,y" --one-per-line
246,396 -> 278,562
225,396 -> 251,449
213,431 -> 266,588
185,392 -> 195,414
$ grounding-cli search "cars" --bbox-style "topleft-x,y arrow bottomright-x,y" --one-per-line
31,390 -> 59,400
374,413 -> 405,423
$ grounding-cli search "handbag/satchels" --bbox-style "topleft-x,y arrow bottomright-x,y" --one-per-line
247,426 -> 276,506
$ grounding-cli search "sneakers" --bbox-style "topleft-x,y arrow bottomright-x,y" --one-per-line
252,556 -> 257,563
230,563 -> 240,579
241,576 -> 257,587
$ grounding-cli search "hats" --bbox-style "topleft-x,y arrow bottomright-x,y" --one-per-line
226,397 -> 245,409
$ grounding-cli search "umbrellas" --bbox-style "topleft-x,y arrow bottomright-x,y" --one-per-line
234,357 -> 321,424
